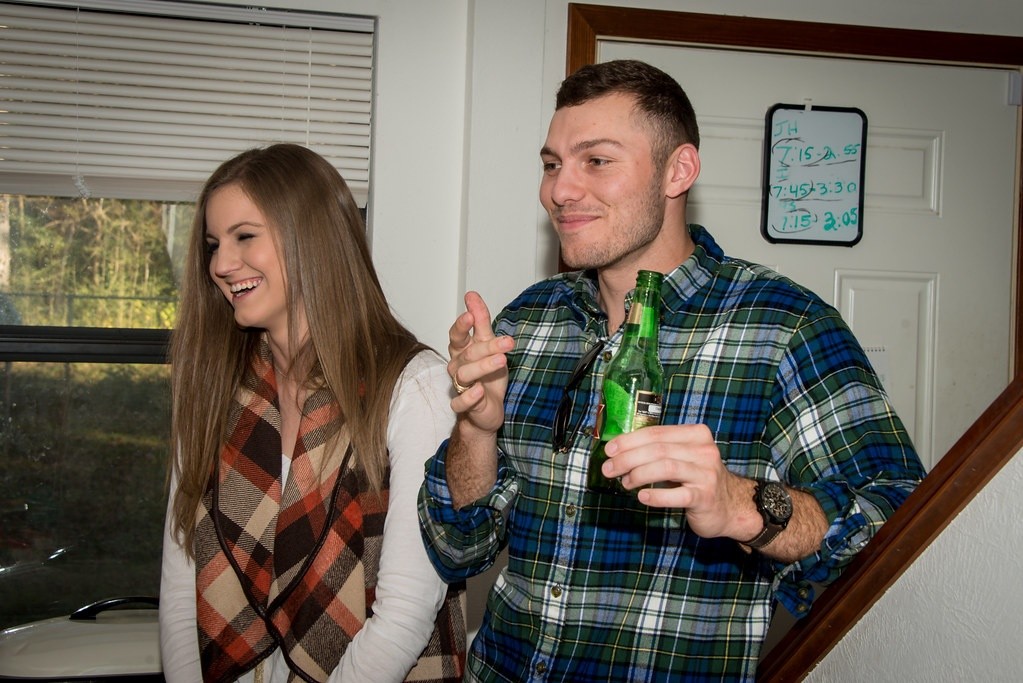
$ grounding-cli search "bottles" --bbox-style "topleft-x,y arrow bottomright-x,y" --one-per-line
587,268 -> 666,496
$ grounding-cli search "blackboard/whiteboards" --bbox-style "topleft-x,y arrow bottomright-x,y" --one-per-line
760,103 -> 869,247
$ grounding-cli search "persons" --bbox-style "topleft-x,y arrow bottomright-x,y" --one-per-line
420,60 -> 926,681
156,140 -> 472,683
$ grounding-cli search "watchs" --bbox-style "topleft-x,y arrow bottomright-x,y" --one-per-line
739,473 -> 795,555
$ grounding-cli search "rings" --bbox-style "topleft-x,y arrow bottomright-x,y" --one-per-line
451,374 -> 471,395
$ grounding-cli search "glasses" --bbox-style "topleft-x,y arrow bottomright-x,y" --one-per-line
552,341 -> 605,454
